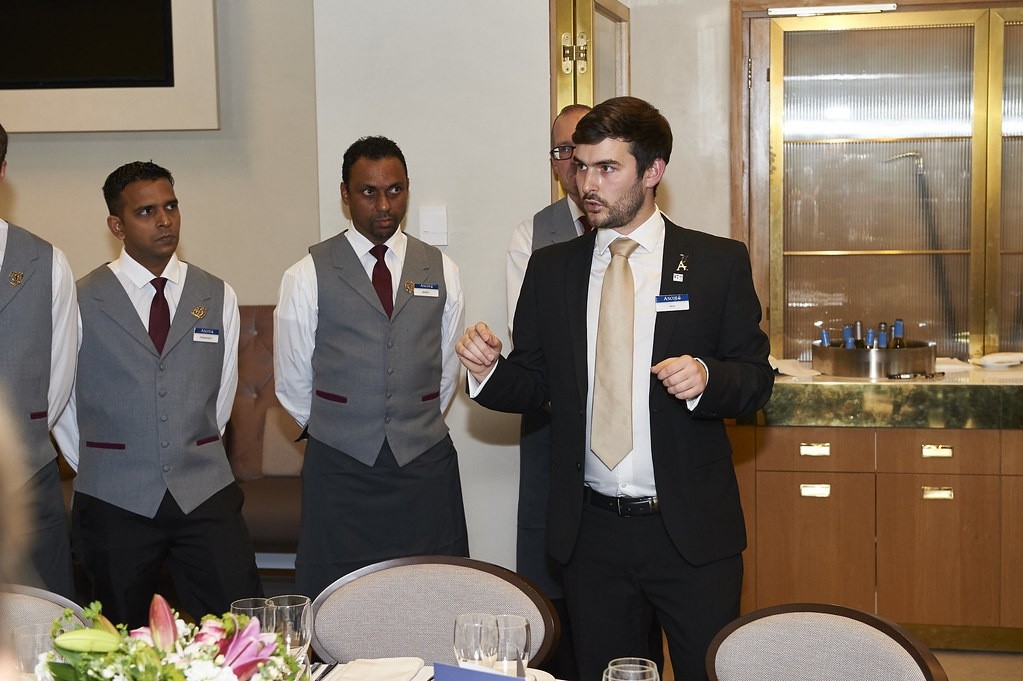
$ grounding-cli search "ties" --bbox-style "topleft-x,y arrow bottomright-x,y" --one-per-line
578,215 -> 593,236
148,277 -> 170,356
368,244 -> 394,321
590,237 -> 640,471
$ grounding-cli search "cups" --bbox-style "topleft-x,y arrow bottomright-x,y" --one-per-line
11,623 -> 62,681
479,615 -> 531,677
264,595 -> 314,668
602,657 -> 660,681
453,613 -> 499,671
230,598 -> 275,635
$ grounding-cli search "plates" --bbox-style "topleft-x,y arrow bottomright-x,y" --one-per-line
522,667 -> 556,681
971,358 -> 1020,369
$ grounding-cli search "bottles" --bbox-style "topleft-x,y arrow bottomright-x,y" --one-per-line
820,319 -> 906,350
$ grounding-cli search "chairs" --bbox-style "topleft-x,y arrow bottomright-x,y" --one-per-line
300,555 -> 560,669
704,602 -> 949,681
0,582 -> 94,672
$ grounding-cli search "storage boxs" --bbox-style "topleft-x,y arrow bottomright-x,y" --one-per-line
811,339 -> 937,377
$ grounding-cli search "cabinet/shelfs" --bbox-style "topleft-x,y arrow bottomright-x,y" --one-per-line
891,421 -> 894,424
724,422 -> 1023,654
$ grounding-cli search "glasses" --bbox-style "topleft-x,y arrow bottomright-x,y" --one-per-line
550,145 -> 577,160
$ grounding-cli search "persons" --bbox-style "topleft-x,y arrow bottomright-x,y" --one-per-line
453,95 -> 775,681
0,126 -> 82,605
51,157 -> 266,632
504,101 -> 592,339
273,137 -> 469,605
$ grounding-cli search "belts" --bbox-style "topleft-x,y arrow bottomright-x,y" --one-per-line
582,487 -> 660,518
